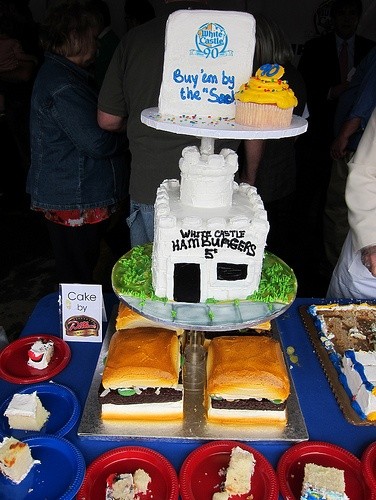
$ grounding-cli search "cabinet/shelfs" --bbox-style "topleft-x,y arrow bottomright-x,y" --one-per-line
77,106 -> 310,449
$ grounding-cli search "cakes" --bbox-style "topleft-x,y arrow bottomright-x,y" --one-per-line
212,445 -> 256,500
308,302 -> 376,422
158,10 -> 256,119
205,335 -> 291,427
149,146 -> 269,305
298,463 -> 349,500
115,302 -> 184,337
0,436 -> 41,485
4,391 -> 51,431
27,338 -> 54,370
106,468 -> 151,500
97,329 -> 185,422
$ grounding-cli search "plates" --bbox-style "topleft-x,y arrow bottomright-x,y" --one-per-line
0,383 -> 82,442
361,441 -> 376,498
277,442 -> 375,500
180,440 -> 278,500
77,445 -> 180,500
0,434 -> 87,500
0,334 -> 71,384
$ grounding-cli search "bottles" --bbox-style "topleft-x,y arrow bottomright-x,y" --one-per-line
182,331 -> 207,393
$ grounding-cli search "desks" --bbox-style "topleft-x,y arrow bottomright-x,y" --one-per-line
0,288 -> 376,500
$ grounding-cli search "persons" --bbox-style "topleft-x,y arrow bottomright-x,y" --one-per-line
95,0 -> 265,251
325,105 -> 376,300
324,42 -> 376,267
84,1 -> 154,95
300,0 -> 376,155
30,17 -> 118,283
241,11 -> 307,202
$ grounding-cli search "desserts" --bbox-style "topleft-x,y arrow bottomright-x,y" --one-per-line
234,63 -> 297,131
